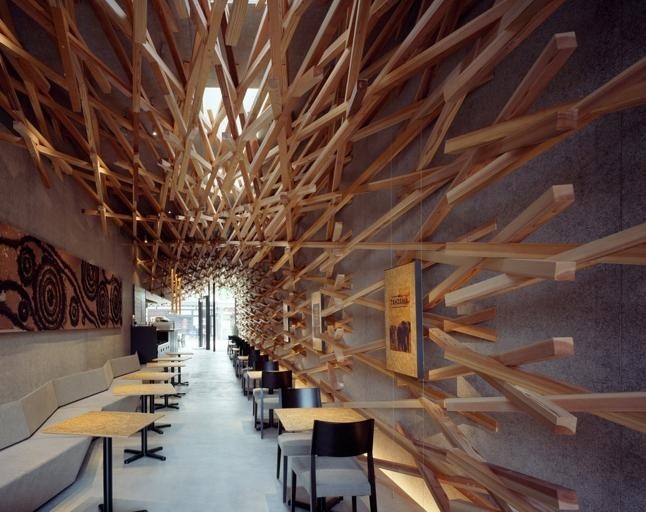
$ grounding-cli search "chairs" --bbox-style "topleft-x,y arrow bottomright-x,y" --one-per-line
228,334 -> 377,512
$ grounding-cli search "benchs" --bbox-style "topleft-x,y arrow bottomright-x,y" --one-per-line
0,350 -> 165,512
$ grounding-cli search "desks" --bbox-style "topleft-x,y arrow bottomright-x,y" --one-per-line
38,349 -> 196,512
